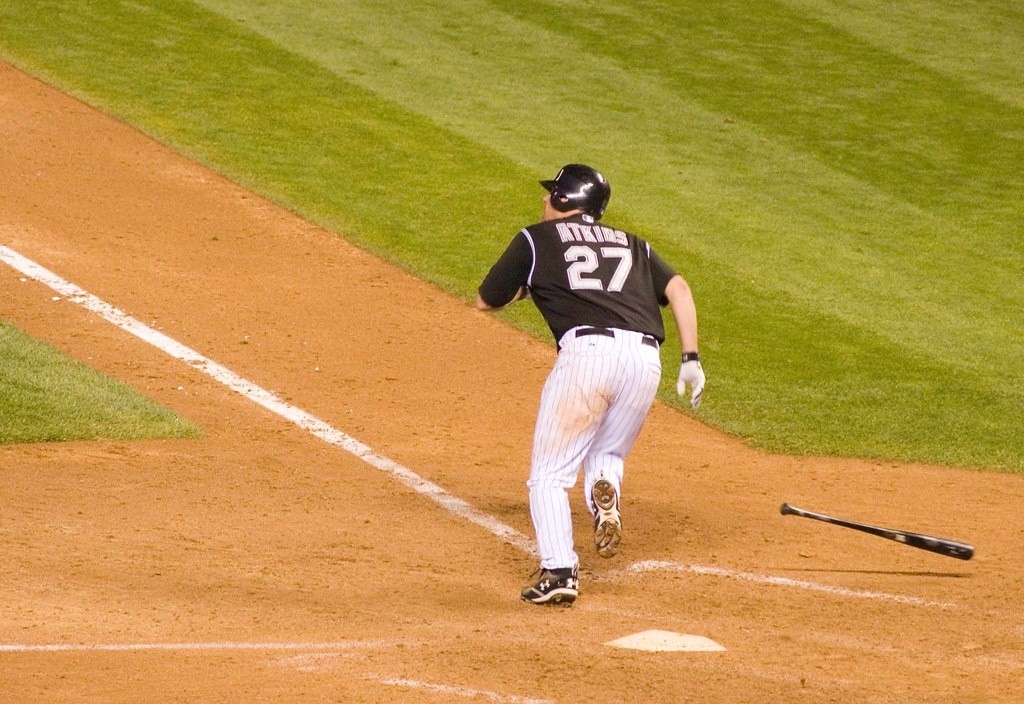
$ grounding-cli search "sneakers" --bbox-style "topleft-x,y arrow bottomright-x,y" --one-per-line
590,470 -> 622,559
521,563 -> 579,605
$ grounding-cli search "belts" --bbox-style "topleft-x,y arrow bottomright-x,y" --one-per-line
557,328 -> 658,354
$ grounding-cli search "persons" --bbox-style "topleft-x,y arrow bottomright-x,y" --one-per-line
476,164 -> 705,608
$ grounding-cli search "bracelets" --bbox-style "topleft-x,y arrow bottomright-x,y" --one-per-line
682,352 -> 699,363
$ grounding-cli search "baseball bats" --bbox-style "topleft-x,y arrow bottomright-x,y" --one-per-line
780,502 -> 975,561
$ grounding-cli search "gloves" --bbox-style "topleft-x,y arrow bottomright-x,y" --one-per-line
678,360 -> 705,409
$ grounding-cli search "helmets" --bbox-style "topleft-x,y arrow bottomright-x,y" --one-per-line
539,164 -> 611,221
